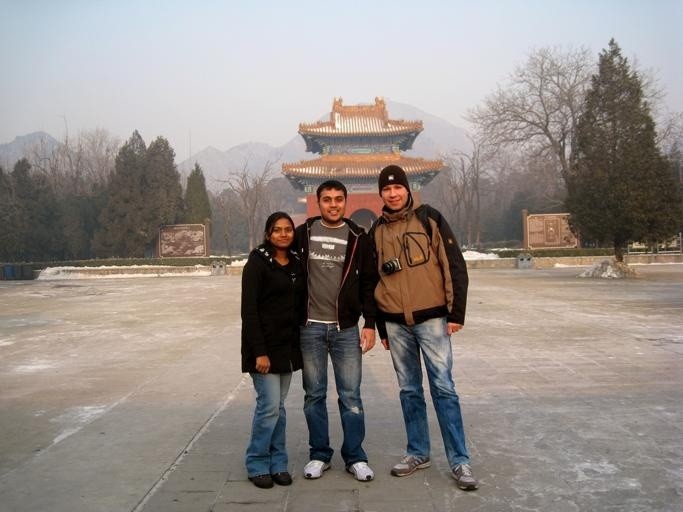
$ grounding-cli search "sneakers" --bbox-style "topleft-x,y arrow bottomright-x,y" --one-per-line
449,460 -> 479,491
346,460 -> 375,481
303,459 -> 333,478
247,469 -> 292,488
389,452 -> 431,477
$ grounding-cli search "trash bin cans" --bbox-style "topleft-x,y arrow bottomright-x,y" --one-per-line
517,253 -> 533,269
211,260 -> 226,276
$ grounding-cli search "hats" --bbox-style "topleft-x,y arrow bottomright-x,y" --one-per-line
378,164 -> 411,198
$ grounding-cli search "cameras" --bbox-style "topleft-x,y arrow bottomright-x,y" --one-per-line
382,258 -> 402,274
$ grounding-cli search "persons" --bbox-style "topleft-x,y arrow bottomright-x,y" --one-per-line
238,211 -> 306,489
367,166 -> 479,491
294,180 -> 375,482
